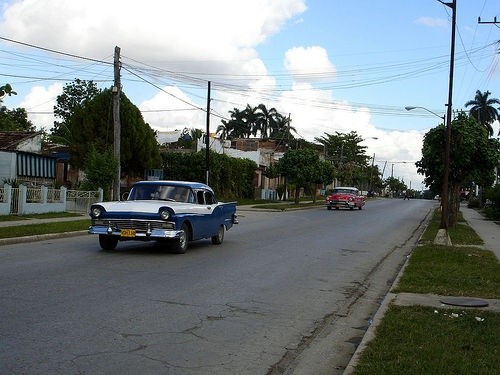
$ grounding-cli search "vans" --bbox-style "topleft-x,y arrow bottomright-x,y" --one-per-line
324,187 -> 359,197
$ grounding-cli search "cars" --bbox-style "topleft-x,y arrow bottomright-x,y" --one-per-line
326,193 -> 365,210
87,180 -> 239,253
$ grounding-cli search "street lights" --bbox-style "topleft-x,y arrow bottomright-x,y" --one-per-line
405,106 -> 446,229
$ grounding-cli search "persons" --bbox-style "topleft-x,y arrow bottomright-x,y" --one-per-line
403,191 -> 409,200
